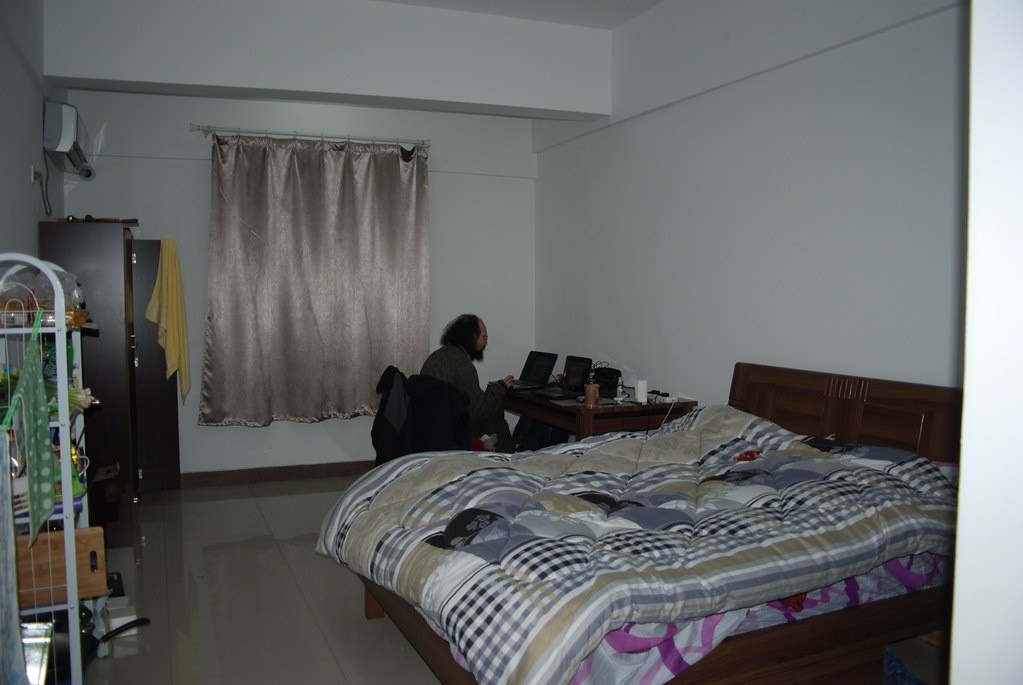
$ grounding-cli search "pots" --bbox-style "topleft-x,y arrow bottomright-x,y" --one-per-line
21,606 -> 149,685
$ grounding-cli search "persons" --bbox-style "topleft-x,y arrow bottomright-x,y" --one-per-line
420,313 -> 514,453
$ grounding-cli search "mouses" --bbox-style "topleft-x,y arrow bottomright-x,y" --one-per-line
576,395 -> 585,403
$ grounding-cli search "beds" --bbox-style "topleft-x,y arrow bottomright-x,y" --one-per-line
313,362 -> 963,685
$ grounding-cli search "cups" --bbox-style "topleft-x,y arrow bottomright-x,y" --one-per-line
584,382 -> 599,406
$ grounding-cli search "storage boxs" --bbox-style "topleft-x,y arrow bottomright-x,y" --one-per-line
634,380 -> 648,403
107,596 -> 138,638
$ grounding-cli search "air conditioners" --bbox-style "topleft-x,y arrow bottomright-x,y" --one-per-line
44,100 -> 91,175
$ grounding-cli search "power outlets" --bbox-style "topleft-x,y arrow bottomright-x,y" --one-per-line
31,166 -> 36,184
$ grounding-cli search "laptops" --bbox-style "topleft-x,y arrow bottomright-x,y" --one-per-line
528,355 -> 593,400
511,351 -> 559,391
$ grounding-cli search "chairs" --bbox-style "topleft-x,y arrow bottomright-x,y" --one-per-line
370,365 -> 497,468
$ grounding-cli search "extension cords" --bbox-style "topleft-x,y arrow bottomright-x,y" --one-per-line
646,392 -> 678,404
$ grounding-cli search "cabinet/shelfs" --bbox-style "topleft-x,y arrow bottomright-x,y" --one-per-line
0,252 -> 92,685
38,217 -> 180,521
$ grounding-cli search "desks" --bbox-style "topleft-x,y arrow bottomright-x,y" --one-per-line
488,382 -> 697,443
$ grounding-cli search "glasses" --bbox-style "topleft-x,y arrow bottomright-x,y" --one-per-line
477,335 -> 488,339
